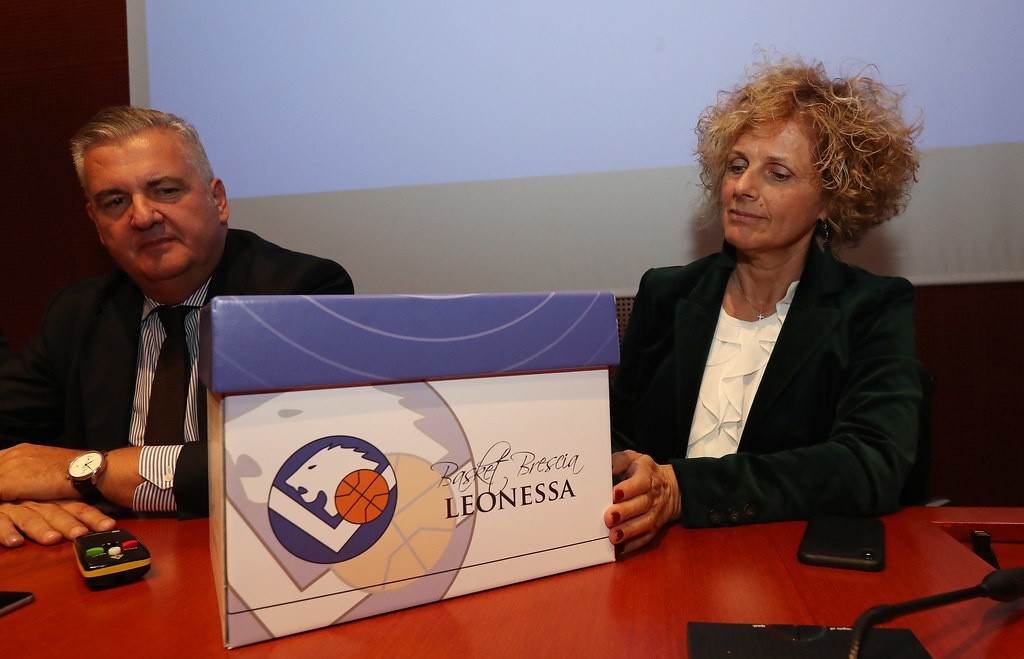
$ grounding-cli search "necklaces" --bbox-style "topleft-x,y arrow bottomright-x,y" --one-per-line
731,270 -> 801,321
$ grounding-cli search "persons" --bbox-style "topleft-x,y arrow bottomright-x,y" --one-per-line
0,105 -> 355,549
604,64 -> 923,556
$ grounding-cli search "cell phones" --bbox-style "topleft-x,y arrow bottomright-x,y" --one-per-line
0,591 -> 36,618
798,514 -> 886,573
71,527 -> 155,590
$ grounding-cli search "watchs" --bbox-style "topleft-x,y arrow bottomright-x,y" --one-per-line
65,448 -> 109,506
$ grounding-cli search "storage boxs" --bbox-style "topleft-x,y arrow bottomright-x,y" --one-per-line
196,289 -> 622,650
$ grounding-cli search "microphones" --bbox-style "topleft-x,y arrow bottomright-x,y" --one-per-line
686,567 -> 1024,659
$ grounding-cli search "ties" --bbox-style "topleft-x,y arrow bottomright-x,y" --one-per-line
143,305 -> 202,445
